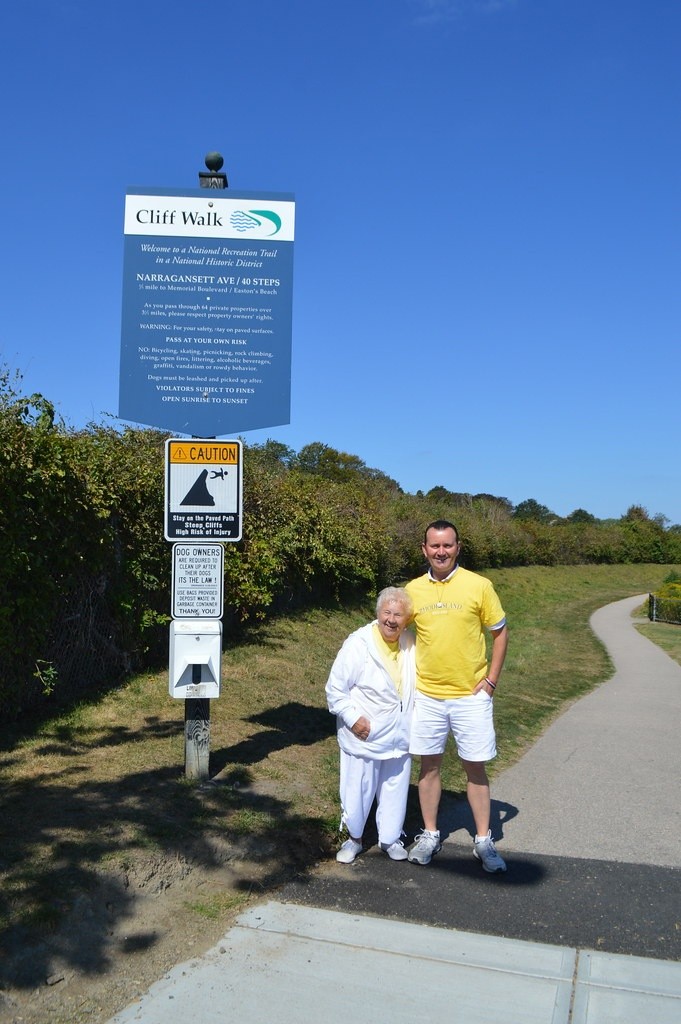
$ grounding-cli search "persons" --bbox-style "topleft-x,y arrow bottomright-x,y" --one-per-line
325,586 -> 417,864
401,521 -> 509,874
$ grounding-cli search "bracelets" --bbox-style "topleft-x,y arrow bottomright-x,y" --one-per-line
485,677 -> 496,689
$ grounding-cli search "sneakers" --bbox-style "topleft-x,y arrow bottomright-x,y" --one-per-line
472,829 -> 508,874
377,839 -> 409,861
336,837 -> 362,863
408,828 -> 441,865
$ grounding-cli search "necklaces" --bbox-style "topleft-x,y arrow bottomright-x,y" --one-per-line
435,582 -> 445,608
